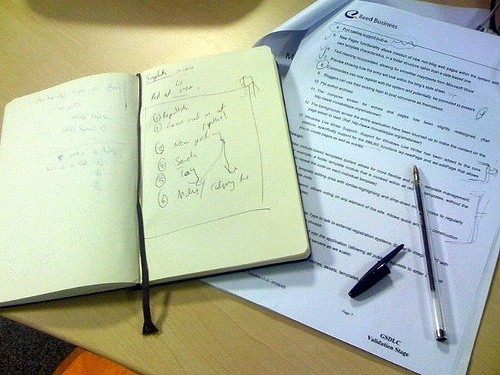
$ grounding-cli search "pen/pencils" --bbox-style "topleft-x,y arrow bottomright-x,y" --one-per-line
412,163 -> 448,340
475,0 -> 500,31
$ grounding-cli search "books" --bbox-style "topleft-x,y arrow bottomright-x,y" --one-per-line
1,45 -> 312,336
